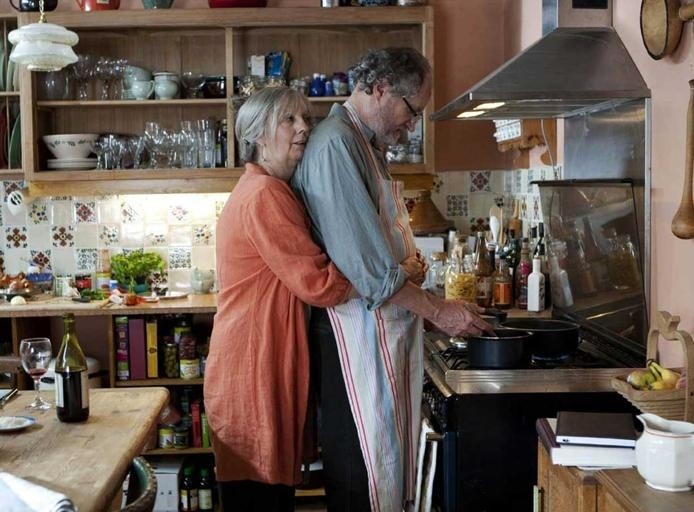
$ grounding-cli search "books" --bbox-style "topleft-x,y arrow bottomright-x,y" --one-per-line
536,417 -> 635,466
555,410 -> 637,447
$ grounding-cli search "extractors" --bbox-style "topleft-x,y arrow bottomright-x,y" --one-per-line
427,26 -> 651,123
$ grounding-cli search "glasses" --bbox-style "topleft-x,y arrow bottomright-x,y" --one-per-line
402,97 -> 422,124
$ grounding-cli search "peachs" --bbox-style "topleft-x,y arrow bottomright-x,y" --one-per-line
675,377 -> 687,392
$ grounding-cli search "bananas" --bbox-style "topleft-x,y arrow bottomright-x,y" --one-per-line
645,358 -> 680,391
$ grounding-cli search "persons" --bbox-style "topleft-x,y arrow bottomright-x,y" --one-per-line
294,46 -> 496,512
202,89 -> 428,512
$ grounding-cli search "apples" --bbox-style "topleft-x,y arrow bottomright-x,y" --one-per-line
626,370 -> 655,393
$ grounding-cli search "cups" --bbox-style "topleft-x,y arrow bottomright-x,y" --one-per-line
131,80 -> 154,100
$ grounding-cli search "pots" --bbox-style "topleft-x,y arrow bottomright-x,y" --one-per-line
498,316 -> 581,357
468,327 -> 533,367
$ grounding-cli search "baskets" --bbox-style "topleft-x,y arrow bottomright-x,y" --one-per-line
611,310 -> 694,424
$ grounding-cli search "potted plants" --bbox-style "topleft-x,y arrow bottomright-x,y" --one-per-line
111,250 -> 165,295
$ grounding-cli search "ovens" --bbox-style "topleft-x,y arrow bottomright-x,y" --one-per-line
422,369 -> 457,512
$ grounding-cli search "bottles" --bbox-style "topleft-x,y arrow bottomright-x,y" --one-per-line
178,336 -> 201,379
54,313 -> 89,422
428,230 -> 549,312
160,336 -> 172,354
180,118 -> 227,169
179,467 -> 214,511
550,214 -> 641,307
164,343 -> 180,378
47,158 -> 98,171
173,314 -> 190,343
96,249 -> 111,296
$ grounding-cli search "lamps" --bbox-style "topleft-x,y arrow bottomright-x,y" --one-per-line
7,0 -> 78,74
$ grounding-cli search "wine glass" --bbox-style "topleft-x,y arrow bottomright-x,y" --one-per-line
181,71 -> 205,98
90,120 -> 180,169
72,55 -> 127,101
20,337 -> 52,413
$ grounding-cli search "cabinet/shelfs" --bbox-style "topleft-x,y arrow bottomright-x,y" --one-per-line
13,313 -> 111,402
0,314 -> 16,395
0,13 -> 28,178
112,312 -> 216,511
429,0 -> 523,173
230,8 -> 428,176
21,7 -> 230,178
532,434 -> 596,512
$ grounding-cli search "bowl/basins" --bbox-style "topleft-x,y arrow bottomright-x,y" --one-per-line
42,134 -> 100,158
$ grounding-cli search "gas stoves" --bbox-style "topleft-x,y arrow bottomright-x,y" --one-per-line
423,329 -> 639,396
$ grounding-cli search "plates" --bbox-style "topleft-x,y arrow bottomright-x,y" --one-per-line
0,415 -> 36,430
